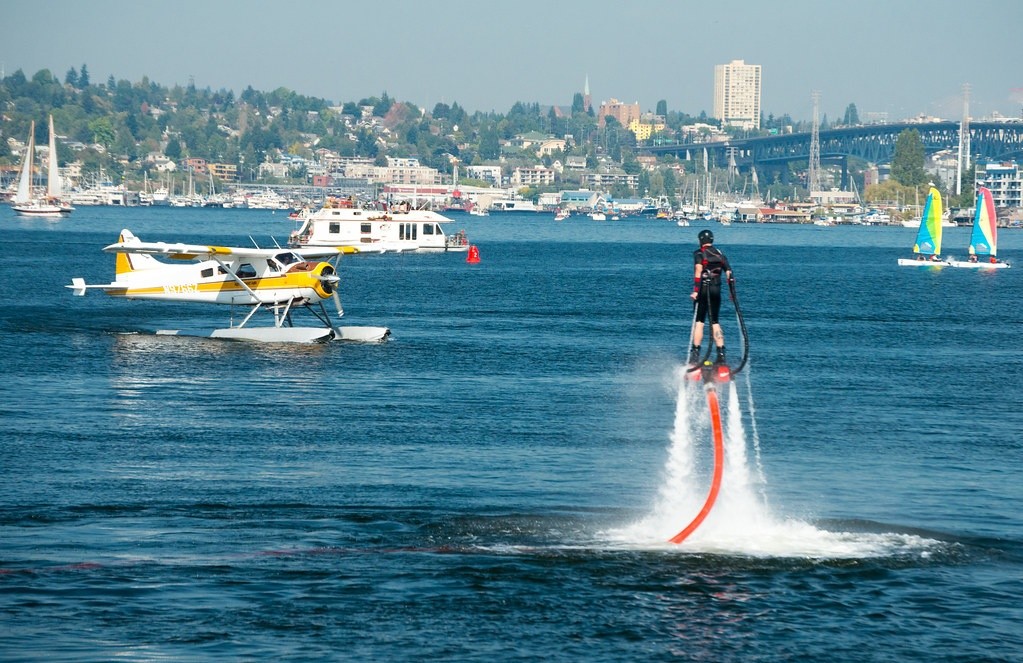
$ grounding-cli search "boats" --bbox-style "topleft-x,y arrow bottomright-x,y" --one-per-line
286,200 -> 471,254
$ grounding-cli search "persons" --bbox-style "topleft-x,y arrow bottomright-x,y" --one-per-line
687,231 -> 734,367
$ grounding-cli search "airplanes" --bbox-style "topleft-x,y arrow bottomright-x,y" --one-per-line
63,226 -> 393,347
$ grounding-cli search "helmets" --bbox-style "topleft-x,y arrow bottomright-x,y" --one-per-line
699,230 -> 713,244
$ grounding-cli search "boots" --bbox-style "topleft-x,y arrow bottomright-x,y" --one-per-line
714,346 -> 725,365
689,344 -> 701,365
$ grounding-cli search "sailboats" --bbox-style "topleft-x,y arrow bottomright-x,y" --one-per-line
896,185 -> 951,268
9,113 -> 76,218
946,185 -> 1011,269
